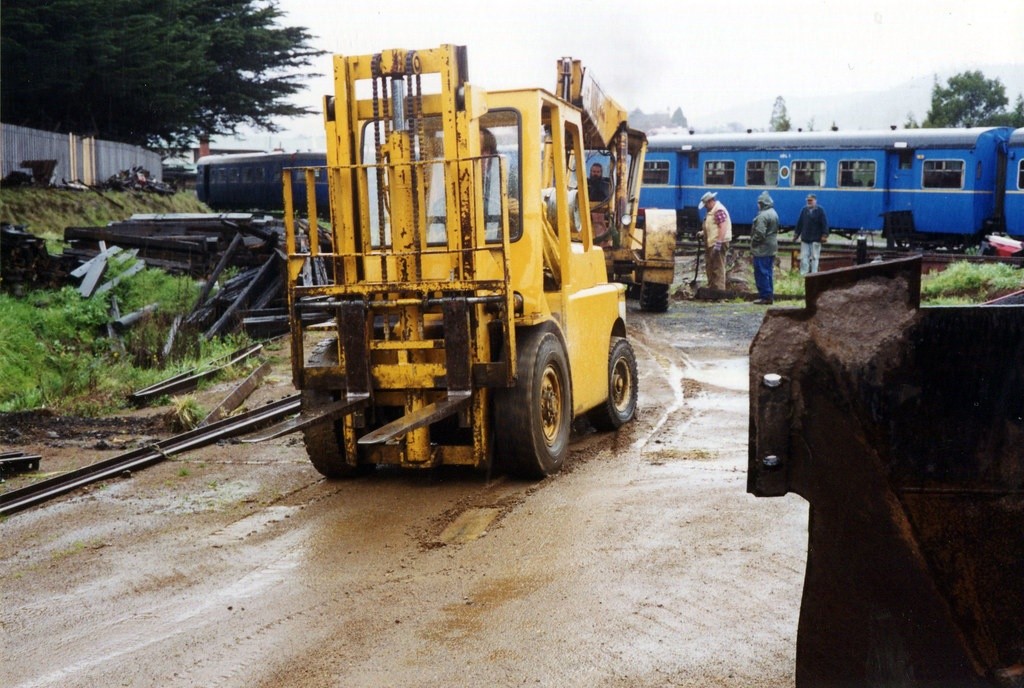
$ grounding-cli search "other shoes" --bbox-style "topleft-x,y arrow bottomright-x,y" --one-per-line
754,298 -> 773,304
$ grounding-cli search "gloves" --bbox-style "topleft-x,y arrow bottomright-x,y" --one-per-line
713,240 -> 721,255
695,231 -> 704,238
507,196 -> 519,214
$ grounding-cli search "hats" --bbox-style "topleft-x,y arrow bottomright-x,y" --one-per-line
806,194 -> 816,200
757,191 -> 773,205
698,192 -> 719,210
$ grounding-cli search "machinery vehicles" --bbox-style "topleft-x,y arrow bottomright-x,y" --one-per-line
540,55 -> 679,312
224,44 -> 639,483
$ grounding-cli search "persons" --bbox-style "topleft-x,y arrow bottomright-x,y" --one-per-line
476,124 -> 520,239
793,194 -> 828,274
750,191 -> 779,305
697,191 -> 732,291
584,161 -> 611,214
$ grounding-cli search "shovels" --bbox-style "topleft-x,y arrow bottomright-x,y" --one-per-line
689,234 -> 702,293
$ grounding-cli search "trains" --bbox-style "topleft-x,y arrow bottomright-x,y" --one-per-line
196,123 -> 1024,254
